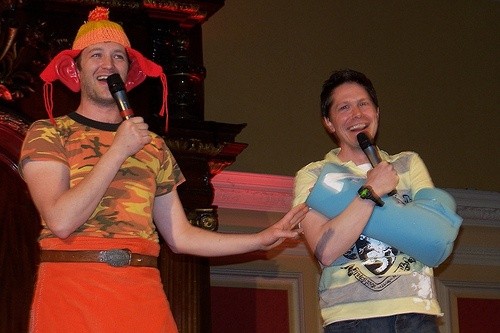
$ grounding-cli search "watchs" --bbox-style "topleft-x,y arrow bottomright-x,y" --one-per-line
358,185 -> 384,207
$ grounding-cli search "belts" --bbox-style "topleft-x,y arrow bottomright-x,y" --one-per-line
39,248 -> 158,268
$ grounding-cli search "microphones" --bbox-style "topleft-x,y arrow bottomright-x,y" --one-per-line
107,73 -> 135,121
358,130 -> 398,196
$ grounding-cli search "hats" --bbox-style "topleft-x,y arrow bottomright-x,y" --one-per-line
40,7 -> 169,131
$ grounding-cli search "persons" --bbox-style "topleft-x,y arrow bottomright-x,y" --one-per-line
17,6 -> 309,333
292,69 -> 464,333
